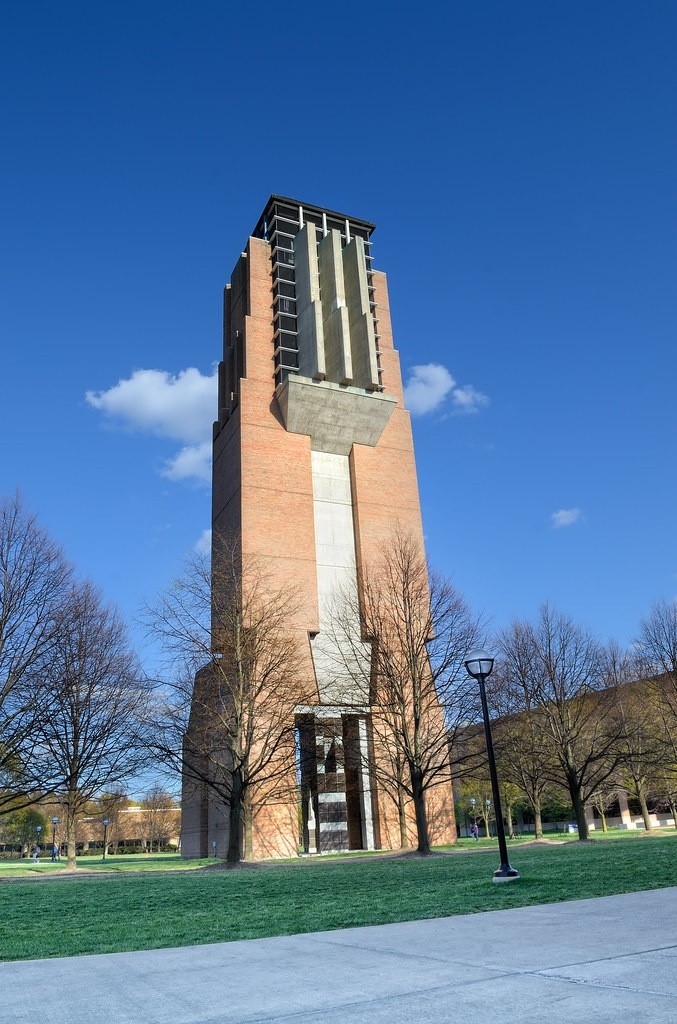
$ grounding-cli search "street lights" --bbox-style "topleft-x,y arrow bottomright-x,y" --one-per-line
51,817 -> 59,862
37,826 -> 41,846
103,819 -> 108,859
463,649 -> 520,885
470,798 -> 477,824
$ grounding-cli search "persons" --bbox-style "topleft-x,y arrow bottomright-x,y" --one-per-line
469,822 -> 479,842
30,840 -> 59,863
